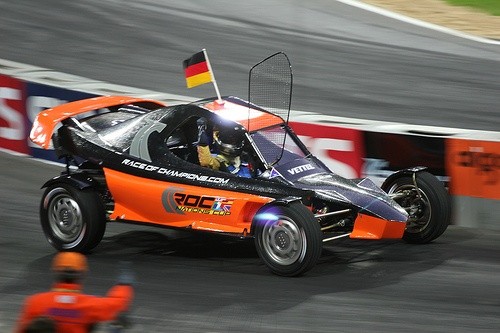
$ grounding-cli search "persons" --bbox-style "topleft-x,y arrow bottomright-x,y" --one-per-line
15,252 -> 135,333
197,119 -> 262,181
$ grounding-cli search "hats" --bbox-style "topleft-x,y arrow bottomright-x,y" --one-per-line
52,251 -> 92,277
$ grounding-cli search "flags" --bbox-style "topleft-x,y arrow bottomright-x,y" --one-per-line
183,51 -> 213,89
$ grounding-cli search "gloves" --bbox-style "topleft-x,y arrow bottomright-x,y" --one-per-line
194,113 -> 212,150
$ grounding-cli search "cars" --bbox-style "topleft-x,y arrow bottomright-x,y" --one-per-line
29,92 -> 455,277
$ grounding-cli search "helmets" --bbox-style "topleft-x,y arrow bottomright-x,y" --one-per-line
212,123 -> 249,157
